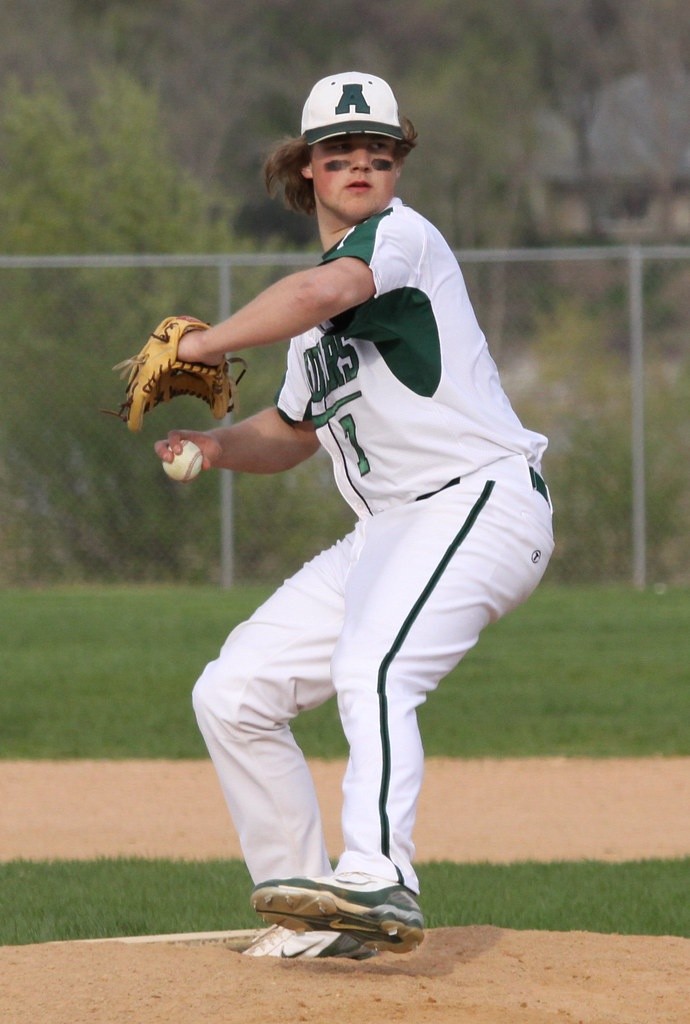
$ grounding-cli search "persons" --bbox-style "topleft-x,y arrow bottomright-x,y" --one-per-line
126,71 -> 555,959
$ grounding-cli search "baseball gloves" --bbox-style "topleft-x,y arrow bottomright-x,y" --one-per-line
126,314 -> 232,436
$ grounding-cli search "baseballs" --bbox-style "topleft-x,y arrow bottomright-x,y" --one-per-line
161,437 -> 205,483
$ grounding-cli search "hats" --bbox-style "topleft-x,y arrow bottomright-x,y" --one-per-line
301,70 -> 404,146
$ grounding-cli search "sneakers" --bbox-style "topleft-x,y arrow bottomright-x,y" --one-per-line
241,921 -> 375,961
250,871 -> 425,954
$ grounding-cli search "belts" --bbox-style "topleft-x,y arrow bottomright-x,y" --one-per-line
415,466 -> 549,505
